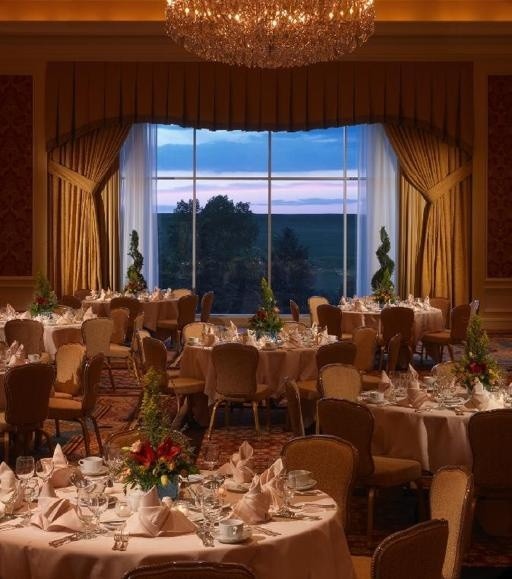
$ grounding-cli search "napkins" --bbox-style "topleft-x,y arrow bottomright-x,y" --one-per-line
259,459 -> 285,506
40,443 -> 71,487
114,485 -> 201,537
27,479 -> 87,534
229,441 -> 256,483
232,473 -> 272,525
1,461 -> 24,518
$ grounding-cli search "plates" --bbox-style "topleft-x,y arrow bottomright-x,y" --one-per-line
81,466 -> 109,476
212,533 -> 252,543
286,479 -> 317,490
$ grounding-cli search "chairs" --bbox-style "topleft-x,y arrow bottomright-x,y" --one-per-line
316,398 -> 422,547
2,286 -> 481,457
280,435 -> 360,530
125,561 -> 257,578
351,466 -> 475,579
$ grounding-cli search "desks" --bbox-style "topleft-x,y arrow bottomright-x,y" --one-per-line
2,464 -> 341,577
353,388 -> 512,474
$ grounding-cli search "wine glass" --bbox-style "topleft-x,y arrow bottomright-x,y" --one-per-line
194,474 -> 229,547
262,470 -> 296,522
0,456 -> 53,528
74,488 -> 155,551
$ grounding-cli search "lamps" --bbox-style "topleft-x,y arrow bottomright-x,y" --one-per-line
163,0 -> 377,71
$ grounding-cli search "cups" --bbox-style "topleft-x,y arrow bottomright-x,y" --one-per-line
78,456 -> 103,473
368,368 -> 511,412
219,520 -> 244,537
289,468 -> 313,485
173,501 -> 189,516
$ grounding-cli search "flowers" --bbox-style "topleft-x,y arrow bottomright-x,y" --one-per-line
127,439 -> 185,487
463,353 -> 491,381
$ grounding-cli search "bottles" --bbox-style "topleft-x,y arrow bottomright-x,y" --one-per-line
162,496 -> 173,507
